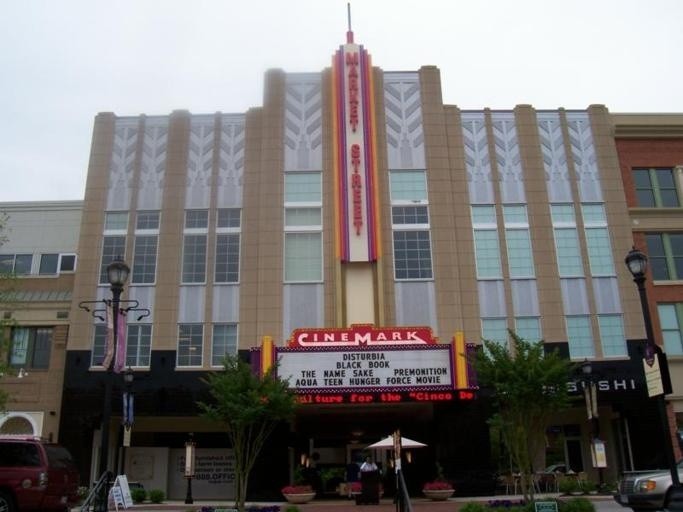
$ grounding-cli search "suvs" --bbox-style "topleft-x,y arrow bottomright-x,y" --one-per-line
609,430 -> 682,512
0,434 -> 80,512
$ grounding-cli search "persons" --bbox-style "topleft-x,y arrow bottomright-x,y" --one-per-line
364,434 -> 428,450
359,454 -> 377,472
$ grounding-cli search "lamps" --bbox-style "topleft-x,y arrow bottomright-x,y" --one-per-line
496,462 -> 571,494
101,254 -> 131,512
299,451 -> 311,469
623,245 -> 680,471
180,429 -> 200,505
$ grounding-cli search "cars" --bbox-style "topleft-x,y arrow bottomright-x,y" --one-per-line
534,463 -> 566,476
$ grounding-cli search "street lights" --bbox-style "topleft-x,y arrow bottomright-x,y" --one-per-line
74,253 -> 154,512
120,360 -> 137,476
622,240 -> 683,510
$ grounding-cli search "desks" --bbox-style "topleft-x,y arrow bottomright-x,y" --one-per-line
361,470 -> 380,505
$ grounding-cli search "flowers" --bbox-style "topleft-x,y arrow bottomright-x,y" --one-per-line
351,481 -> 384,492
281,485 -> 314,493
424,480 -> 453,489
